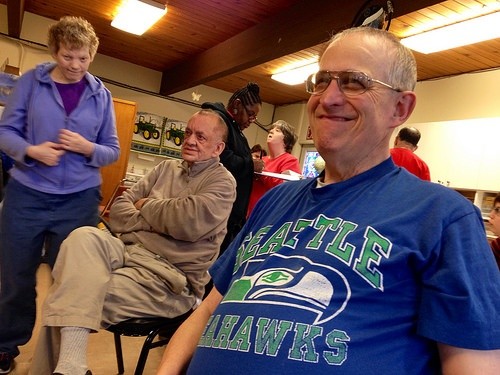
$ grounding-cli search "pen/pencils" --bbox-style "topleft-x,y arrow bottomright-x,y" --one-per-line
260,150 -> 262,159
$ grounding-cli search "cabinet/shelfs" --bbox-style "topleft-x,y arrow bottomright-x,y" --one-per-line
292,101 -> 325,179
438,186 -> 500,239
87,97 -> 137,215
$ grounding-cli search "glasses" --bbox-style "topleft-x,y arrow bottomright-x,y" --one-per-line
243,104 -> 257,120
305,70 -> 401,97
493,204 -> 500,216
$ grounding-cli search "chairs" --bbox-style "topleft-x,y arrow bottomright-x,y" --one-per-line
101,204 -> 246,375
98,178 -> 137,216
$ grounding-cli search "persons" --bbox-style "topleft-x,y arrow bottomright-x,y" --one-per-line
155,27 -> 500,375
201,83 -> 265,260
389,127 -> 430,182
245,120 -> 302,220
250,144 -> 267,160
487,196 -> 500,272
0,16 -> 120,374
28,111 -> 237,375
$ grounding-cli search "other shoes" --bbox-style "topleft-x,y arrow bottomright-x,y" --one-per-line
0,352 -> 13,373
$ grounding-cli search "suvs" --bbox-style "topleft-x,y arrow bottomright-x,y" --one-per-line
134,116 -> 160,140
166,123 -> 185,146
